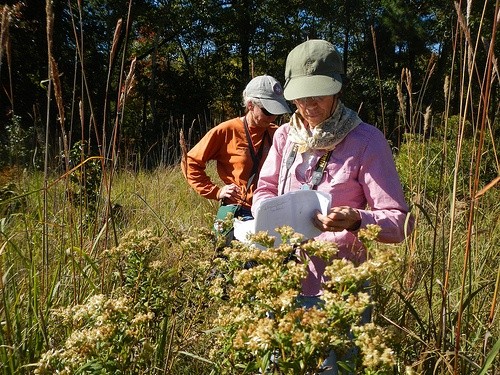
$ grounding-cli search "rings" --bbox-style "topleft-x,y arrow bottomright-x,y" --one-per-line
330,227 -> 334,231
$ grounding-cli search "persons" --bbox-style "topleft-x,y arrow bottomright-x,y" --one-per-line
180,75 -> 292,270
250,39 -> 415,375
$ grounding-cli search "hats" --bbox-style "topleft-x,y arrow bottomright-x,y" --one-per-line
283,37 -> 344,100
246,76 -> 292,115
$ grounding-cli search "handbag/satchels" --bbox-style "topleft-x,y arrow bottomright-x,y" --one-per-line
212,204 -> 242,245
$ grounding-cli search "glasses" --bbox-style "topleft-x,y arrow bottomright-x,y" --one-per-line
254,102 -> 273,116
